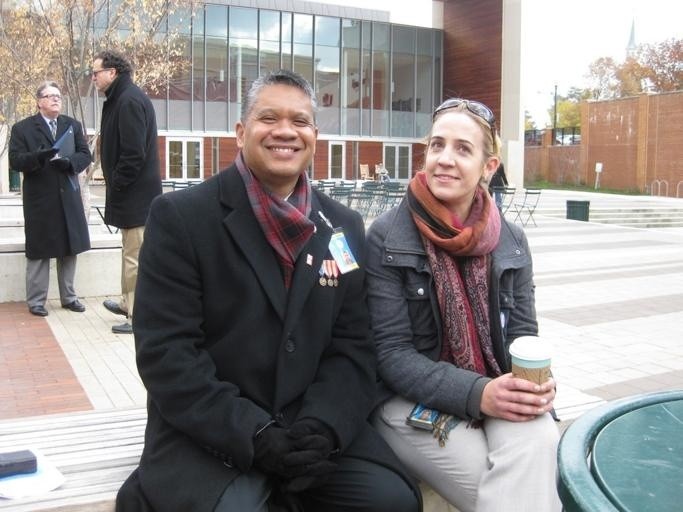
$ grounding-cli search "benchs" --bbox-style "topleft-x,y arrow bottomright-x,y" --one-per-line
0,384 -> 605,511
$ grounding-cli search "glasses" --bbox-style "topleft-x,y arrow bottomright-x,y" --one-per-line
432,97 -> 496,141
41,93 -> 62,98
90,68 -> 110,78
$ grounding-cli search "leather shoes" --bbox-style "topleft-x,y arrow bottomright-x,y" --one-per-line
62,300 -> 85,312
111,324 -> 134,334
28,305 -> 47,316
103,299 -> 128,319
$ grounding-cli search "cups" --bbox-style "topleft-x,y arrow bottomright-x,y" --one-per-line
509,336 -> 552,385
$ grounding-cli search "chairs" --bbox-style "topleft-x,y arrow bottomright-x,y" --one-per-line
494,185 -> 543,229
309,179 -> 406,222
360,164 -> 375,181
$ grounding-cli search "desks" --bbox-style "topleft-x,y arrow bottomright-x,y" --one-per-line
90,206 -> 120,233
556,388 -> 683,511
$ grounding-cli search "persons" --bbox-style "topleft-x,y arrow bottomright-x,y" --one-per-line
364,95 -> 565,512
375,162 -> 391,184
114,70 -> 424,509
90,51 -> 162,334
8,81 -> 91,316
489,162 -> 508,218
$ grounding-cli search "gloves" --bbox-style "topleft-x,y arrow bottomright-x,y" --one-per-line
37,144 -> 59,161
251,418 -> 339,494
52,157 -> 71,171
